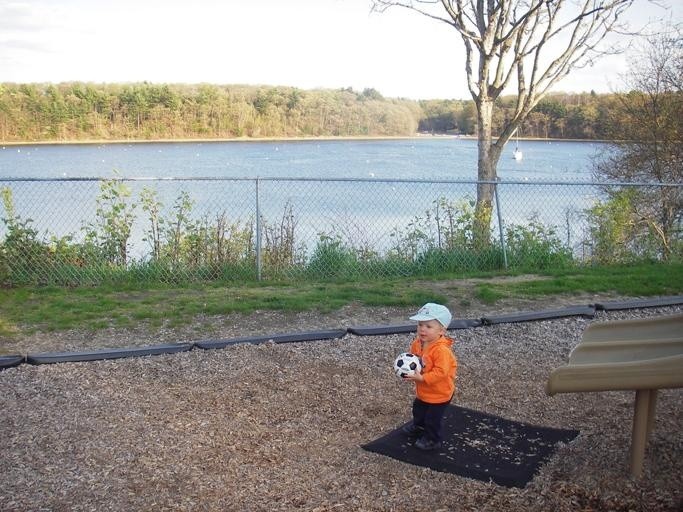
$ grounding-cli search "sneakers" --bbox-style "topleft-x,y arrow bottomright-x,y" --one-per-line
403,422 -> 442,450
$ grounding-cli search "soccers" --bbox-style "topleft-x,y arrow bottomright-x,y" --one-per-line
393,351 -> 422,379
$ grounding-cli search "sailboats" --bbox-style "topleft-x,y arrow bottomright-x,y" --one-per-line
513,129 -> 523,161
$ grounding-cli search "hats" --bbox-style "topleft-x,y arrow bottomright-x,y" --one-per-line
409,303 -> 451,329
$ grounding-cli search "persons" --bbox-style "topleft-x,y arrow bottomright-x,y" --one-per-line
402,302 -> 458,450
